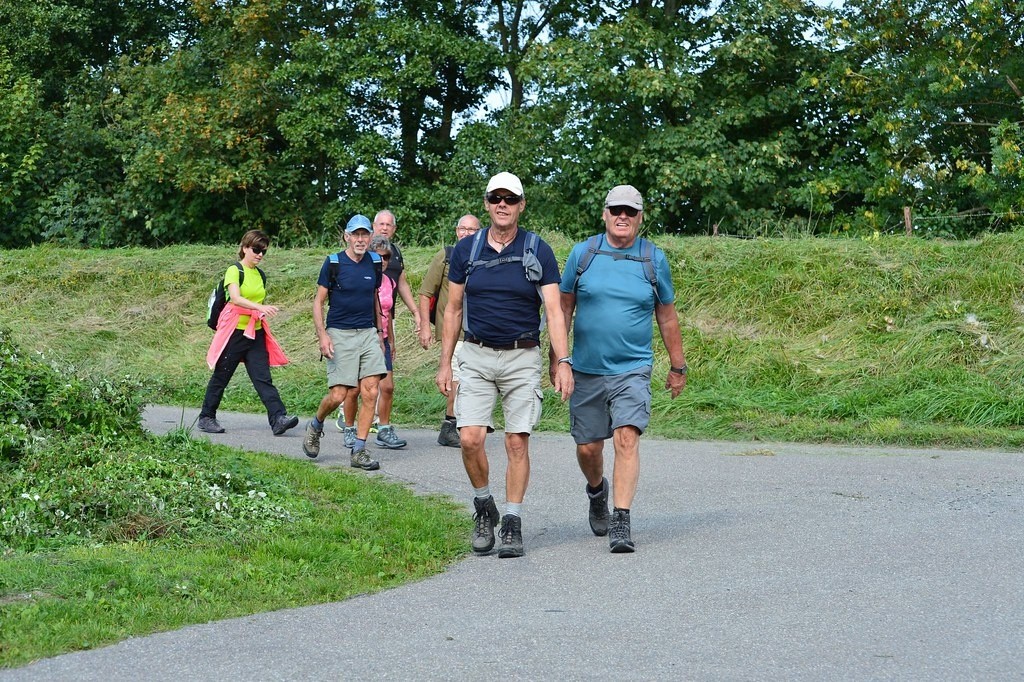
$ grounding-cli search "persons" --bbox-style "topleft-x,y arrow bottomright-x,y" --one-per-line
419,215 -> 481,447
302,214 -> 388,470
335,210 -> 421,432
197,229 -> 299,435
436,171 -> 574,557
344,235 -> 407,447
548,185 -> 686,552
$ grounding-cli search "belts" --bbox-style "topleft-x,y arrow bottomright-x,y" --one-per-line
466,335 -> 538,351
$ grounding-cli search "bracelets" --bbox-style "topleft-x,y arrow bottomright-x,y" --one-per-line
377,329 -> 383,333
412,309 -> 417,314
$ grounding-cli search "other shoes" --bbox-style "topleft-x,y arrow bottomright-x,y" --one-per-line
369,421 -> 379,434
336,407 -> 346,431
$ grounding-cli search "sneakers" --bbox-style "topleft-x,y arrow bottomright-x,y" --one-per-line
344,427 -> 357,448
437,418 -> 462,447
586,476 -> 610,536
272,413 -> 300,435
609,512 -> 635,553
472,495 -> 500,555
198,414 -> 225,433
303,419 -> 325,457
350,449 -> 379,470
375,427 -> 407,448
497,513 -> 523,558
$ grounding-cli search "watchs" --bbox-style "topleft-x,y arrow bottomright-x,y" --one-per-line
558,357 -> 573,365
671,365 -> 687,374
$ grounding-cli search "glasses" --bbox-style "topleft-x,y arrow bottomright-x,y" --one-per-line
487,194 -> 524,206
457,227 -> 480,236
249,245 -> 267,257
377,253 -> 392,262
606,204 -> 639,217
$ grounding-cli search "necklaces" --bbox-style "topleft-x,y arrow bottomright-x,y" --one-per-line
490,229 -> 517,252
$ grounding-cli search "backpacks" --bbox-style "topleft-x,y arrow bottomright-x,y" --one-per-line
207,262 -> 266,330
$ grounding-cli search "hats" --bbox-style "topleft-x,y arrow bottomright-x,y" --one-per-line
605,184 -> 644,212
487,170 -> 525,199
347,215 -> 375,234
